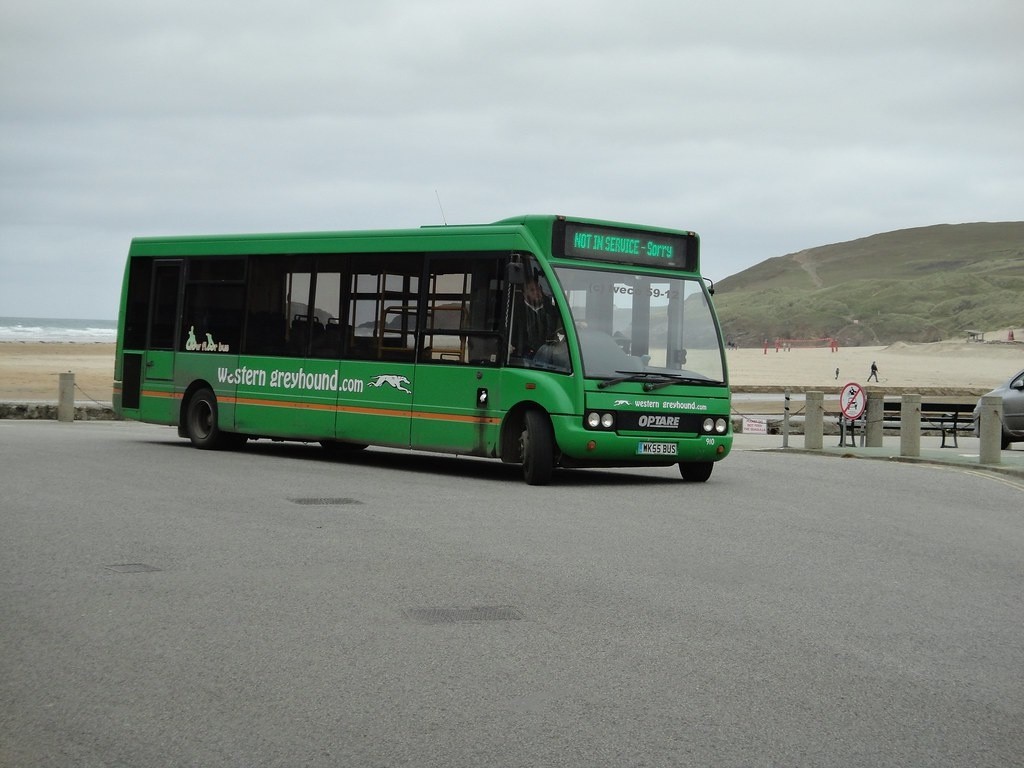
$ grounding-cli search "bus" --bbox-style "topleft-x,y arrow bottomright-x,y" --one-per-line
112,215 -> 734,488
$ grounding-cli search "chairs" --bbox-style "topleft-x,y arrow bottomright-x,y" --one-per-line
203,306 -> 404,359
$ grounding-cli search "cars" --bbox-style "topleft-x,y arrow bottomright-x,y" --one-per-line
973,370 -> 1024,451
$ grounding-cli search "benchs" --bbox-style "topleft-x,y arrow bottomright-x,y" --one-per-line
836,401 -> 977,448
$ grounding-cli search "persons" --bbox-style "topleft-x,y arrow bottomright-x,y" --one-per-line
500,279 -> 562,361
867,361 -> 879,382
835,368 -> 839,379
